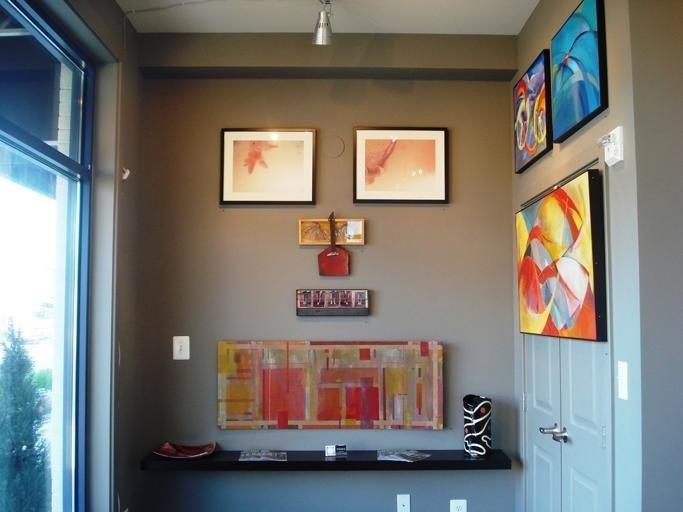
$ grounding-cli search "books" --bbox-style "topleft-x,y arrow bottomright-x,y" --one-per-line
238,450 -> 288,462
376,449 -> 407,461
391,451 -> 432,462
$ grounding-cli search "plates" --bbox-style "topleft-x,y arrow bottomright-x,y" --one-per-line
152,439 -> 216,458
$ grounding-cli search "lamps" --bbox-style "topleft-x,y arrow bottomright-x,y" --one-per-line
308,0 -> 337,49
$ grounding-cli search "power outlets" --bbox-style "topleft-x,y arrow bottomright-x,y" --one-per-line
395,492 -> 466,512
171,336 -> 190,361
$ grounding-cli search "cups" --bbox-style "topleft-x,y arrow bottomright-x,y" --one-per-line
299,292 -> 364,307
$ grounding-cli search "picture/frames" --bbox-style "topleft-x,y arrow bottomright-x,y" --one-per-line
299,218 -> 365,246
352,127 -> 449,204
295,289 -> 371,317
219,339 -> 444,431
511,0 -> 608,343
218,128 -> 319,206
